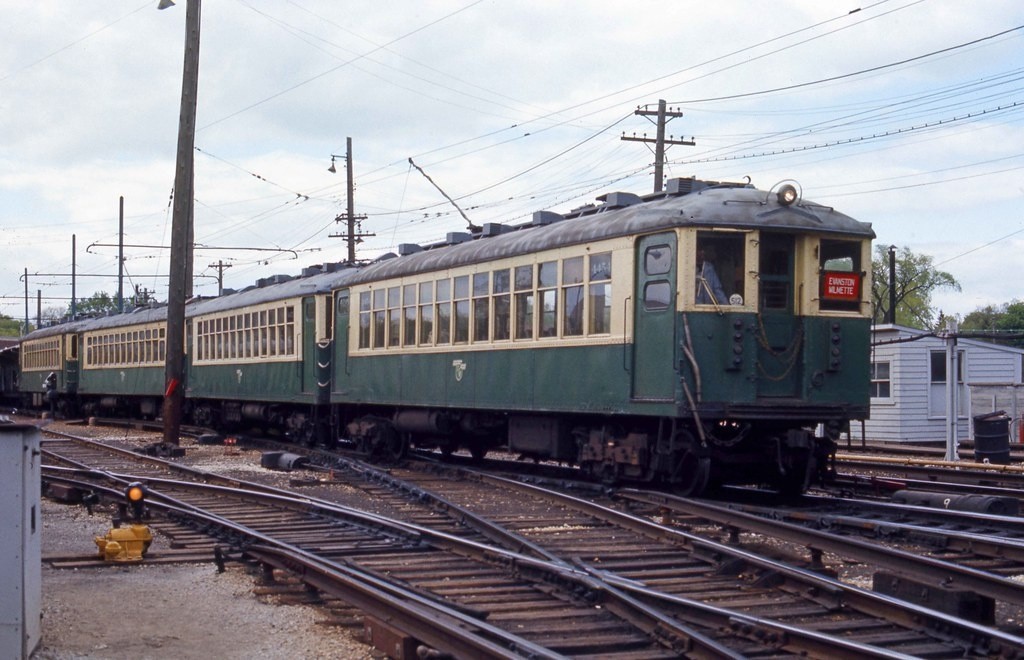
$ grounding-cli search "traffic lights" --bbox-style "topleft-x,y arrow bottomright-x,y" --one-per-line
124,481 -> 151,508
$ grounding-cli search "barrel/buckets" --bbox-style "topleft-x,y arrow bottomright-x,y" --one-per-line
973,415 -> 1010,465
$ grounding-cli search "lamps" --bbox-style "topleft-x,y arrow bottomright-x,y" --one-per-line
124,481 -> 149,525
44,372 -> 57,390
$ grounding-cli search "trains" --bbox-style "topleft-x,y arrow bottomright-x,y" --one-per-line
1,174 -> 878,502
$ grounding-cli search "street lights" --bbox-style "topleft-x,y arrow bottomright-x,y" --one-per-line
328,136 -> 357,262
157,0 -> 204,448
